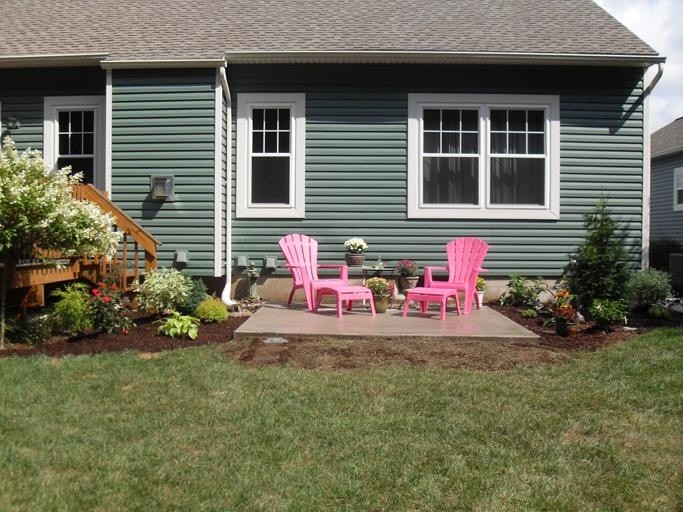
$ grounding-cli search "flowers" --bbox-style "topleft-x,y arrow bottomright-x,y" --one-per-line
552,290 -> 579,319
475,276 -> 487,291
344,238 -> 367,254
395,259 -> 418,277
365,276 -> 392,297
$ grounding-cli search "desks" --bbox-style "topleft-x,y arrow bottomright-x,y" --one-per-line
361,266 -> 396,307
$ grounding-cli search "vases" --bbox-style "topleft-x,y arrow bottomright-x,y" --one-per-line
345,252 -> 365,266
374,294 -> 387,313
472,290 -> 485,311
399,276 -> 419,288
555,317 -> 569,337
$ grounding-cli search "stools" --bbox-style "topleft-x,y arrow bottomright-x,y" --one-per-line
315,286 -> 377,318
401,287 -> 462,322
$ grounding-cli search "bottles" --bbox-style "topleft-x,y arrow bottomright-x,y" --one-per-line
375,255 -> 384,269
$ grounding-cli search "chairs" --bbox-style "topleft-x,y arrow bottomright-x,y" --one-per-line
423,237 -> 489,315
278,233 -> 348,310
